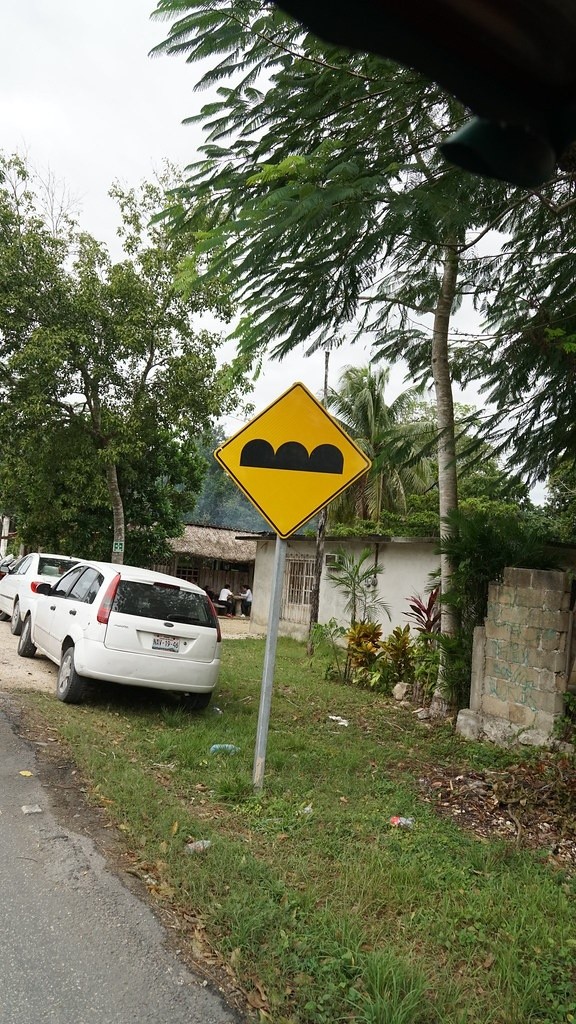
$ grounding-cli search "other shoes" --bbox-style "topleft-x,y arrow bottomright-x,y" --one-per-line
238,614 -> 245,618
227,614 -> 232,618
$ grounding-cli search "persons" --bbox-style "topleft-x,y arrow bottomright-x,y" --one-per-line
204,585 -> 215,602
238,585 -> 252,618
191,577 -> 199,586
217,584 -> 234,617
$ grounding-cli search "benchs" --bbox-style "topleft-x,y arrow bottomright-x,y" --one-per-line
212,602 -> 227,616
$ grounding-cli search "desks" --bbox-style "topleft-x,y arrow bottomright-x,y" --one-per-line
230,596 -> 246,619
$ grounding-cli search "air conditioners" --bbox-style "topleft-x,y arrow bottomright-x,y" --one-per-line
325,554 -> 343,568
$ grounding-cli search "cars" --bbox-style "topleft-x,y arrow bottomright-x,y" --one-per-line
17,561 -> 223,709
0,553 -> 86,635
